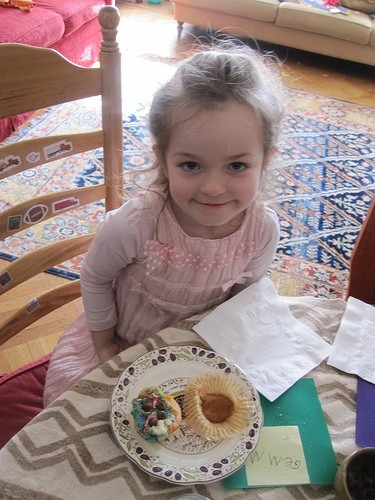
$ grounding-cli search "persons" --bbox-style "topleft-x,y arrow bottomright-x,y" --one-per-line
43,42 -> 287,409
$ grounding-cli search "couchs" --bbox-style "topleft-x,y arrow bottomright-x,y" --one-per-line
172,0 -> 375,66
0,0 -> 115,142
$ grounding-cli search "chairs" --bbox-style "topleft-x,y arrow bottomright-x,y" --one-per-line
0,5 -> 131,447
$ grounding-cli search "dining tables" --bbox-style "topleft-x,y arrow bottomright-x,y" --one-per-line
0,295 -> 375,500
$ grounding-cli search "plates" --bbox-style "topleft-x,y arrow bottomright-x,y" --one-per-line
109,345 -> 263,485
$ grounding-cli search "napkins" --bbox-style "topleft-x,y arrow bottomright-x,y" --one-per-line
326,296 -> 375,384
191,277 -> 334,402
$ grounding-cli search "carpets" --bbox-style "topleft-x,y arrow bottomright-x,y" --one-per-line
0,49 -> 374,282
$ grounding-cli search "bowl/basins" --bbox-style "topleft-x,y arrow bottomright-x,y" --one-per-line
335,447 -> 375,500
